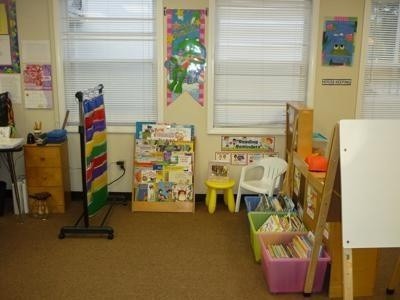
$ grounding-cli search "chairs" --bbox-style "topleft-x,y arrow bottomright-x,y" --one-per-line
235,156 -> 290,214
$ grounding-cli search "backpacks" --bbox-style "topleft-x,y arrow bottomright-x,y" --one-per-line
0,92 -> 14,126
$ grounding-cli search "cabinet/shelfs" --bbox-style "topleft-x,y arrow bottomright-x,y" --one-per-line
23,140 -> 72,216
131,134 -> 197,216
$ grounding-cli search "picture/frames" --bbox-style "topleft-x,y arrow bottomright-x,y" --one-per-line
207,159 -> 231,182
0,1 -> 21,74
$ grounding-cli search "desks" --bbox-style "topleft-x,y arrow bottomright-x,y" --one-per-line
284,101 -> 400,300
1,138 -> 24,219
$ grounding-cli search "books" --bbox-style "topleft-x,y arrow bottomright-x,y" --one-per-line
252,194 -> 326,261
133,123 -> 194,203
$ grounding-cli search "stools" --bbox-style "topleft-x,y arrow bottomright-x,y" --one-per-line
205,178 -> 237,215
